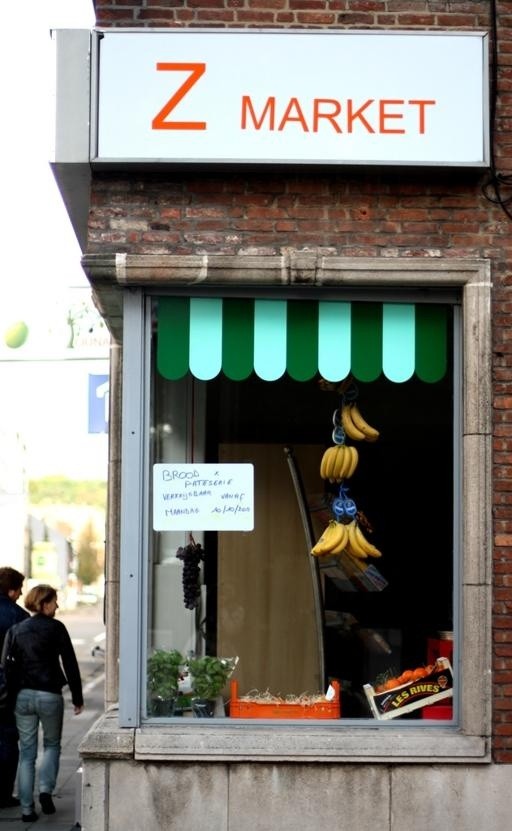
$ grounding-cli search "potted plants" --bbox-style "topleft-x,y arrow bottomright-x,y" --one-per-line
147,649 -> 188,717
184,656 -> 232,717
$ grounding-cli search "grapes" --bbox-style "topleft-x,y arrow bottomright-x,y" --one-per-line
176,544 -> 207,610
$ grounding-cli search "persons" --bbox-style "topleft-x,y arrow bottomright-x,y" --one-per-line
0,583 -> 86,824
1,566 -> 31,808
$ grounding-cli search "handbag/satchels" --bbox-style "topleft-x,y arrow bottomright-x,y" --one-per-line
4,624 -> 18,689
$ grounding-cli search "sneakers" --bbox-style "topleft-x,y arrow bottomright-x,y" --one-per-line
38,793 -> 56,816
22,812 -> 39,823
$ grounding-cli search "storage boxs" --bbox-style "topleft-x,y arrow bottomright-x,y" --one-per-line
362,658 -> 454,720
230,678 -> 340,719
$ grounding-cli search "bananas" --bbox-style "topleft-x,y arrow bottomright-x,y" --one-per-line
345,521 -> 383,559
311,518 -> 348,557
319,444 -> 360,483
340,402 -> 380,443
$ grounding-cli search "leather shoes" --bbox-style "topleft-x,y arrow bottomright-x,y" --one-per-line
0,795 -> 21,810
